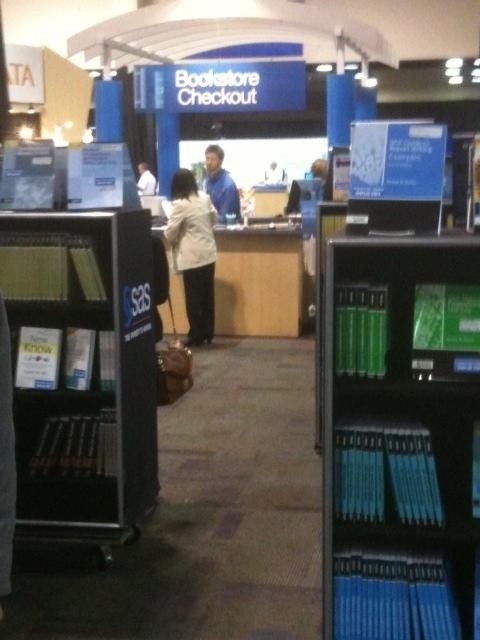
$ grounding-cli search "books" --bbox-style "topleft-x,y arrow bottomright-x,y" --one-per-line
332,276 -> 479,381
331,119 -> 480,237
334,418 -> 479,527
332,548 -> 480,640
14,325 -> 117,391
162,198 -> 182,221
1,139 -> 141,212
29,406 -> 118,478
1,231 -> 109,301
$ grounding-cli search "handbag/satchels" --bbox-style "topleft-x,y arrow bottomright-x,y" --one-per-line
155,341 -> 193,406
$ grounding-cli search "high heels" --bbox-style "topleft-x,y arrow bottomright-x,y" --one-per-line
183,335 -> 202,346
203,334 -> 214,344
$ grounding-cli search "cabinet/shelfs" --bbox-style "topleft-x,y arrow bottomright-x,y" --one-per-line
322,235 -> 477,640
0,207 -> 161,553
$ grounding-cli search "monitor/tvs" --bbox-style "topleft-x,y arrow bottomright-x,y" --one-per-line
285,179 -> 320,214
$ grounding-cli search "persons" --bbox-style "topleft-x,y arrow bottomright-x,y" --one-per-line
159,168 -> 222,345
200,145 -> 240,224
135,161 -> 158,197
149,232 -> 172,344
300,156 -> 329,310
